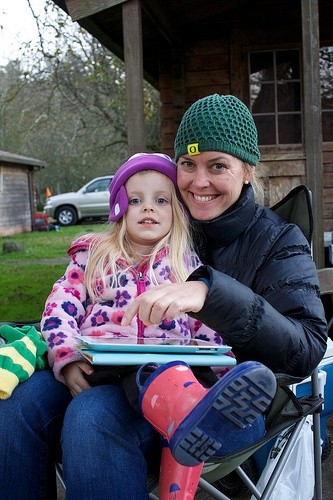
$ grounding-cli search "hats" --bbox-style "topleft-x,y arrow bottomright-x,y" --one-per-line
109,152 -> 177,223
174,93 -> 260,167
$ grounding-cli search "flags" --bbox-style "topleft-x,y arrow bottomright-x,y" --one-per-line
46,188 -> 52,197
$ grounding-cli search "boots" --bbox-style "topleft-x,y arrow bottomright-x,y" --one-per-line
136,360 -> 277,466
159,441 -> 204,500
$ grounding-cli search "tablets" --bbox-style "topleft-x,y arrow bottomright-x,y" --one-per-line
75,336 -> 232,353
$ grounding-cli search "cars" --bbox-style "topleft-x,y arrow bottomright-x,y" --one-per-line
44,175 -> 117,227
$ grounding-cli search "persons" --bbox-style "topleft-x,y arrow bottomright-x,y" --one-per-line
34,203 -> 60,231
40,152 -> 277,500
0,94 -> 328,500
